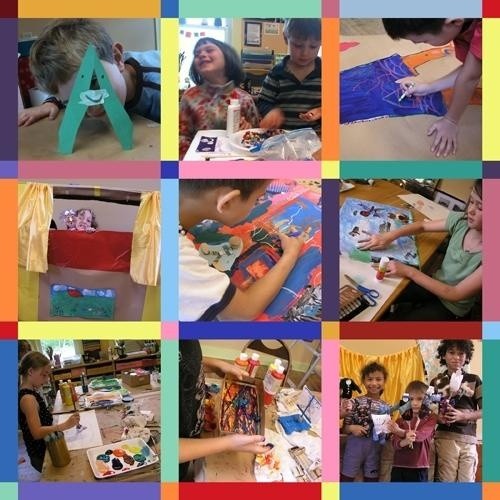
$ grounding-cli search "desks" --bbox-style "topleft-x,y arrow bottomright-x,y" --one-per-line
182,130 -> 321,161
189,372 -> 321,482
39,373 -> 161,482
339,179 -> 459,322
18,101 -> 160,160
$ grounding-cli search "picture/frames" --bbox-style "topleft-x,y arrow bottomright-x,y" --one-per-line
431,188 -> 467,212
264,23 -> 280,35
245,21 -> 262,46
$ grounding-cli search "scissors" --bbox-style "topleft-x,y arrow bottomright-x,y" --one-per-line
344,274 -> 379,306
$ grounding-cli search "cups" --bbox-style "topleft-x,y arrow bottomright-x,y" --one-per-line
42,430 -> 72,468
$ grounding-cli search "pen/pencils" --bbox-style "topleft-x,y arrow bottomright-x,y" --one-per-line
397,82 -> 416,102
276,230 -> 302,242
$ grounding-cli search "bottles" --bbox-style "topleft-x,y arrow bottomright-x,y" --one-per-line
57,377 -> 78,405
224,99 -> 240,135
80,373 -> 88,391
234,351 -> 285,408
152,367 -> 161,383
376,255 -> 389,281
143,340 -> 159,353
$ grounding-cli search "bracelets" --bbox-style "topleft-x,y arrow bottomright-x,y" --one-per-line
444,116 -> 458,128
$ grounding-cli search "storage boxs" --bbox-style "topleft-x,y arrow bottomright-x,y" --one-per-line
121,372 -> 150,387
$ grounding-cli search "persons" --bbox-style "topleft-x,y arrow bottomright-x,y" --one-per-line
256,17 -> 321,131
179,36 -> 260,160
18,342 -> 57,413
357,180 -> 483,321
339,338 -> 483,483
17,17 -> 160,128
17,351 -> 81,473
382,18 -> 482,158
65,207 -> 98,233
179,339 -> 274,481
178,179 -> 305,326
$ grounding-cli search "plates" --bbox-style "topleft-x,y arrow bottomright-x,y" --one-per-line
87,436 -> 159,478
229,127 -> 289,151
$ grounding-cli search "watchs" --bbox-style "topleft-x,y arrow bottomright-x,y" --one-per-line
41,95 -> 63,110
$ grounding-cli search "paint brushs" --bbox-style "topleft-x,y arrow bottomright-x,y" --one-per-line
73,399 -> 82,430
151,435 -> 161,460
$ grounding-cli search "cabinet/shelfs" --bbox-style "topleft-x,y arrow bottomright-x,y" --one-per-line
241,47 -> 275,90
48,350 -> 161,395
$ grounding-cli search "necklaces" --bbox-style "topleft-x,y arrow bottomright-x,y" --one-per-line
179,222 -> 187,235
